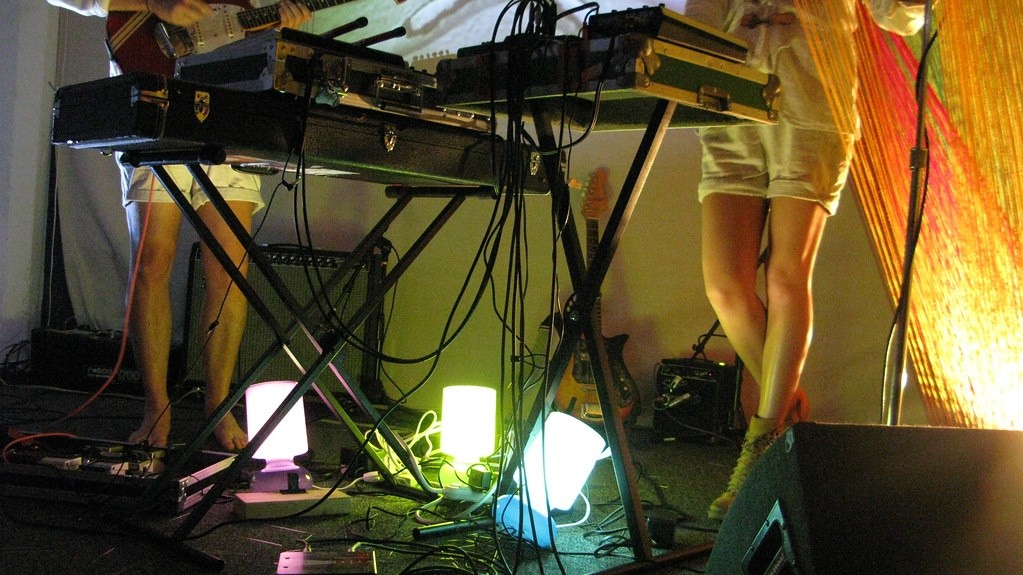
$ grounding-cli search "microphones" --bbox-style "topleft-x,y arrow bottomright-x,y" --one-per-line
655,393 -> 679,405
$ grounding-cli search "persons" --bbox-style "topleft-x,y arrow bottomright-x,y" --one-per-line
48,0 -> 314,453
697,0 -> 935,520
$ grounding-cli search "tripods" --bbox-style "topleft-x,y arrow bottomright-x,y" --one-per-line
660,250 -> 773,458
318,236 -> 437,423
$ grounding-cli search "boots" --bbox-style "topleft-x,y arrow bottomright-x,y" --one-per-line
707,389 -> 809,520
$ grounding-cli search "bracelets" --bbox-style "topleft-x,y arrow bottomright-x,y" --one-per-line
145,0 -> 150,13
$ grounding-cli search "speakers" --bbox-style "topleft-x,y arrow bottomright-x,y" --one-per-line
704,421 -> 1023,575
181,243 -> 383,406
652,359 -> 738,440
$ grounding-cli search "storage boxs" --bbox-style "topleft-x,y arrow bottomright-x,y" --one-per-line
0,432 -> 240,515
48,74 -> 568,191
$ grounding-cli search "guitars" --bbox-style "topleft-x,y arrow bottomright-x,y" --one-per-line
105,0 -> 360,78
548,165 -> 643,425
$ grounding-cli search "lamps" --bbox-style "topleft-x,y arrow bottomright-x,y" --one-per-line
439,385 -> 497,487
491,411 -> 606,548
246,381 -> 312,491
